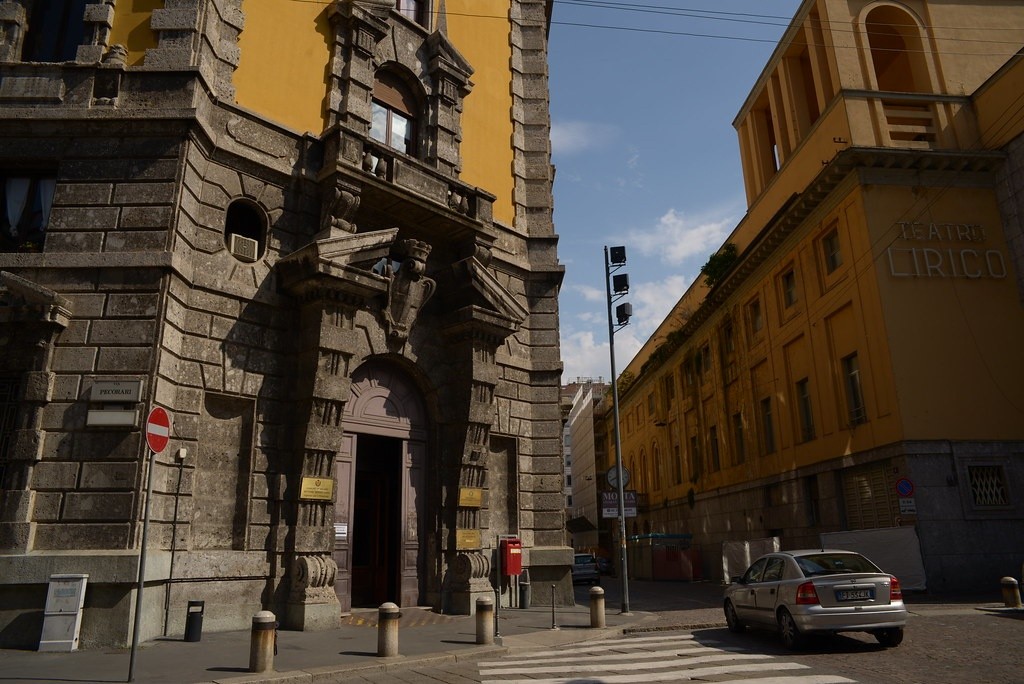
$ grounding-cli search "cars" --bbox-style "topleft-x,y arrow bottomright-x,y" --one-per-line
722,548 -> 908,649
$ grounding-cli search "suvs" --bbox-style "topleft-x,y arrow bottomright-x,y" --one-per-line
571,553 -> 601,586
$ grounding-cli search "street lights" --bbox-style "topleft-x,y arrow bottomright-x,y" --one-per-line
603,244 -> 633,611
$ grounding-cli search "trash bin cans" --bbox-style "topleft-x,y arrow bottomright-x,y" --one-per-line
183,600 -> 205,642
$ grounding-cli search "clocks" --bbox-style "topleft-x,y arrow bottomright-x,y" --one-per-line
607,465 -> 631,490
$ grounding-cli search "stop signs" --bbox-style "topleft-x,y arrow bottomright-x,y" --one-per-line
145,406 -> 171,455
